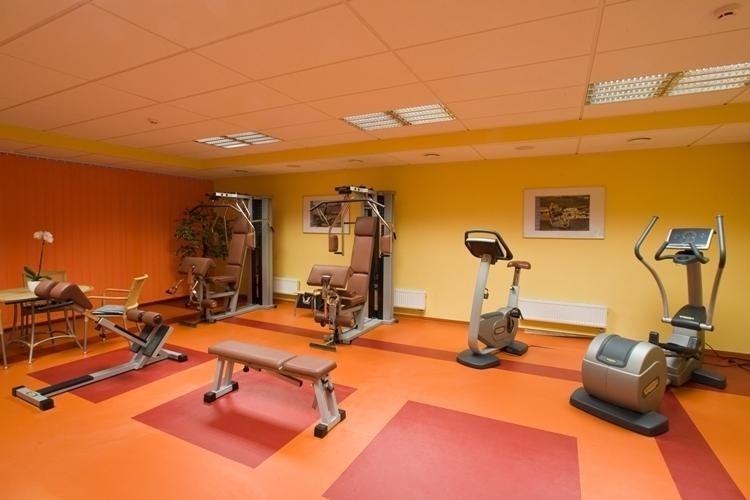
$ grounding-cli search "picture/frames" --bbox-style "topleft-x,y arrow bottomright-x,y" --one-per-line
300,195 -> 350,234
521,187 -> 606,239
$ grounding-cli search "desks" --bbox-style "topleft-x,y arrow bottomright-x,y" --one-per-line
0,284 -> 95,364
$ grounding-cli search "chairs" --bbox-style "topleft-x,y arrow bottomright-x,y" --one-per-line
19,269 -> 68,347
83,273 -> 149,355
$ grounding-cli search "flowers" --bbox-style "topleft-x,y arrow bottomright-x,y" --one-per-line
24,231 -> 56,281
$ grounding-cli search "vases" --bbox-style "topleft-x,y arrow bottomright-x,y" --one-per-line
27,281 -> 39,292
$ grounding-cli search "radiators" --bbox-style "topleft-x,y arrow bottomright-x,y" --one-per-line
517,299 -> 610,329
274,278 -> 299,298
392,289 -> 427,311
